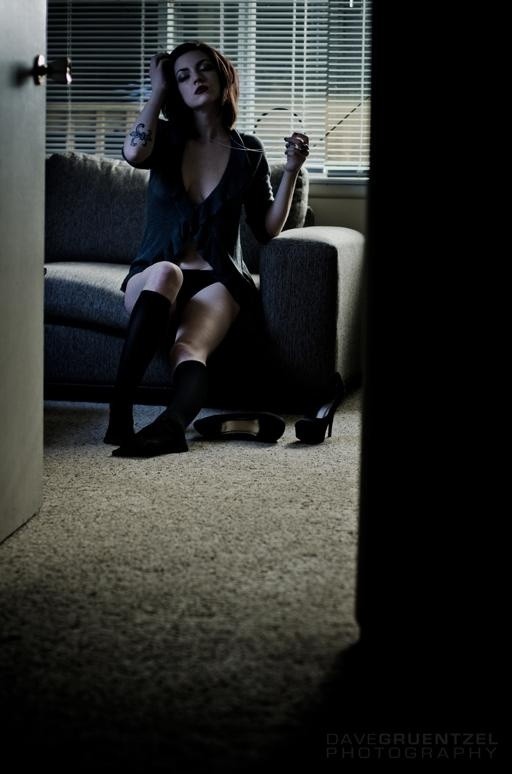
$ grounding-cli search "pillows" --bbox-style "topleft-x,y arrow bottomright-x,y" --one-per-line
47,150 -> 149,261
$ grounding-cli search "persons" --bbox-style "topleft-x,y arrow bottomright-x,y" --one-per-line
102,41 -> 311,461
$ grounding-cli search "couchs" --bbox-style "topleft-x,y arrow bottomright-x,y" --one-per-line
45,197 -> 367,414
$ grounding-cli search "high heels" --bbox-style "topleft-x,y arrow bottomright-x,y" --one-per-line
193,412 -> 285,441
295,372 -> 342,444
